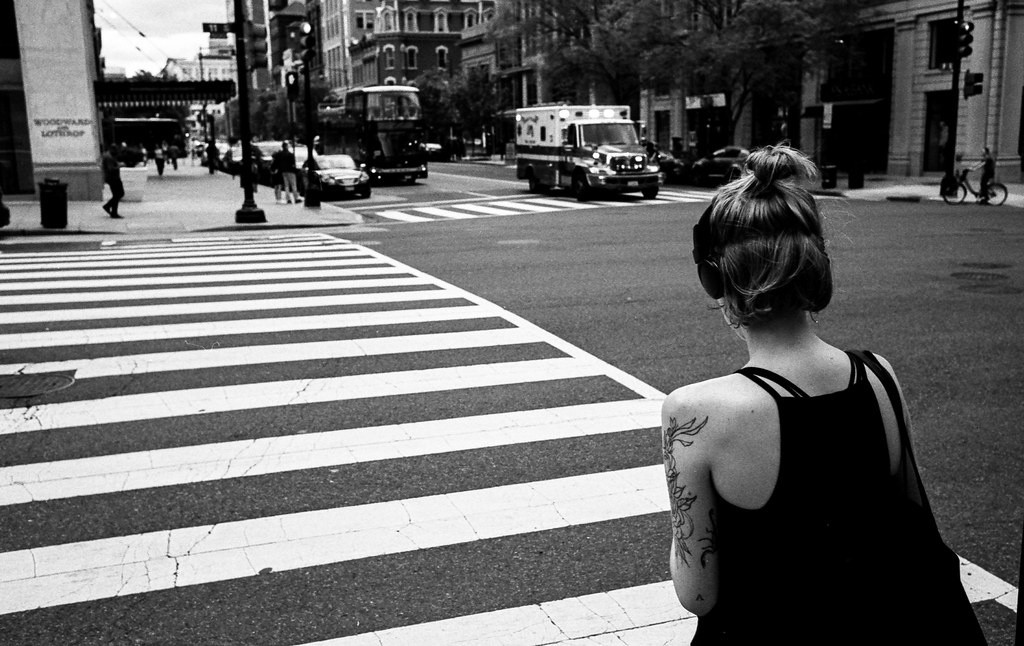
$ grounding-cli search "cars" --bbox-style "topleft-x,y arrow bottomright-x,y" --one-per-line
288,146 -> 319,182
692,146 -> 752,184
189,137 -> 243,174
655,150 -> 689,186
251,140 -> 285,181
301,154 -> 371,201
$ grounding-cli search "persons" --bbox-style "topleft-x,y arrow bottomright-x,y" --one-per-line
936,121 -> 949,172
660,145 -> 915,646
192,149 -> 195,159
973,147 -> 995,205
162,140 -> 179,170
270,140 -> 302,204
101,143 -> 125,218
140,144 -> 148,166
154,143 -> 165,178
250,136 -> 264,192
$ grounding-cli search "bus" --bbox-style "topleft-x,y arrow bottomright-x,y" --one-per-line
342,86 -> 428,186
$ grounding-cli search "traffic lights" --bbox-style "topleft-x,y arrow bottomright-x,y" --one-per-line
963,68 -> 984,100
247,20 -> 268,68
285,72 -> 299,86
292,22 -> 316,62
956,21 -> 974,58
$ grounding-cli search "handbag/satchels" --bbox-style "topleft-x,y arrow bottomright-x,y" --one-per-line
851,349 -> 988,646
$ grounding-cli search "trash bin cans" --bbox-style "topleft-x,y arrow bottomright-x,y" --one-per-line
821,165 -> 836,189
38,178 -> 69,229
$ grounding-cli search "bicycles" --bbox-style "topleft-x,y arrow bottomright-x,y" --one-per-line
942,168 -> 1008,207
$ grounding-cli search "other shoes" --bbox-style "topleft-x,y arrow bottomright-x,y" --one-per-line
102,205 -> 111,214
110,213 -> 125,218
288,200 -> 292,203
276,201 -> 285,205
295,199 -> 303,203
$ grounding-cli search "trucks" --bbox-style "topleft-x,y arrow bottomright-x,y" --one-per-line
514,105 -> 660,201
